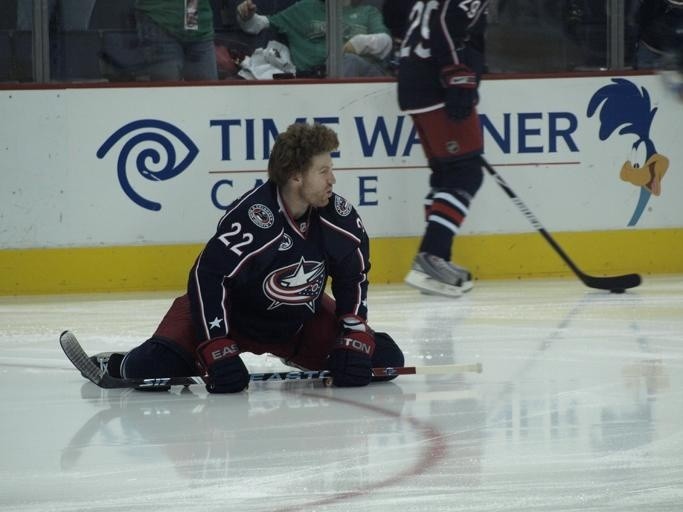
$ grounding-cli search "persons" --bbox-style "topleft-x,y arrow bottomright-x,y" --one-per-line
0,1 -> 297,90
79,125 -> 404,395
397,1 -> 488,298
636,0 -> 683,70
237,1 -> 392,81
562,0 -> 634,71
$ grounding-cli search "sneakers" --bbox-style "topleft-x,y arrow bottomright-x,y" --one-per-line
82,354 -> 125,378
412,252 -> 470,286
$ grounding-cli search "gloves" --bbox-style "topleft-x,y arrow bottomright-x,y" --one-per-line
439,64 -> 479,120
328,330 -> 375,387
196,336 -> 248,394
